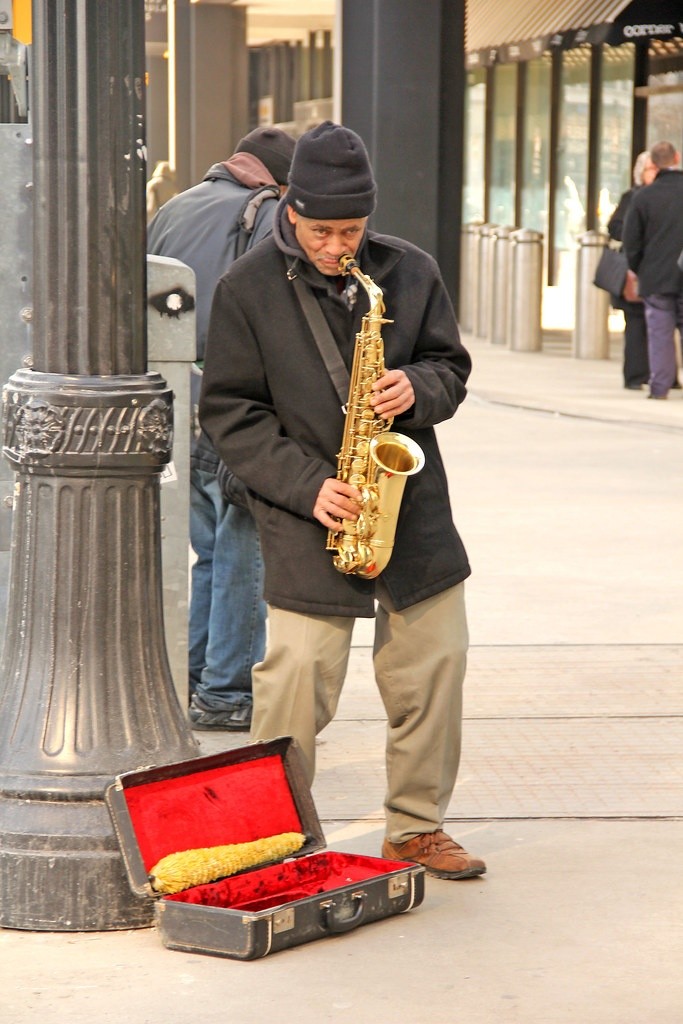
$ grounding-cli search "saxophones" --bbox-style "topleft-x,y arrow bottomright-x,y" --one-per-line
325,253 -> 426,581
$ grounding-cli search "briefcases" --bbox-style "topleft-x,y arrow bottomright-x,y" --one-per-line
103,733 -> 427,962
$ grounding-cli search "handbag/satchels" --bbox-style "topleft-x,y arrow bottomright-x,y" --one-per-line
592,243 -> 628,298
217,458 -> 251,513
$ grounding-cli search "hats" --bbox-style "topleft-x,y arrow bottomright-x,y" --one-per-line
286,120 -> 378,219
234,126 -> 298,186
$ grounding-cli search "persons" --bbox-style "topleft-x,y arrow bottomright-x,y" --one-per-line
147,125 -> 297,731
146,162 -> 180,222
608,150 -> 680,389
200,121 -> 485,880
622,142 -> 683,399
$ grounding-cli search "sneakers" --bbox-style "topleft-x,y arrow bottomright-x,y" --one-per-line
381,827 -> 487,880
188,692 -> 252,732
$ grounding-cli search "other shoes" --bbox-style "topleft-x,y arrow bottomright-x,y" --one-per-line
671,381 -> 682,389
625,382 -> 642,390
648,392 -> 667,399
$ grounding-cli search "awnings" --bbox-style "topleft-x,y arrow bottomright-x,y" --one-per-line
467,0 -> 682,70
